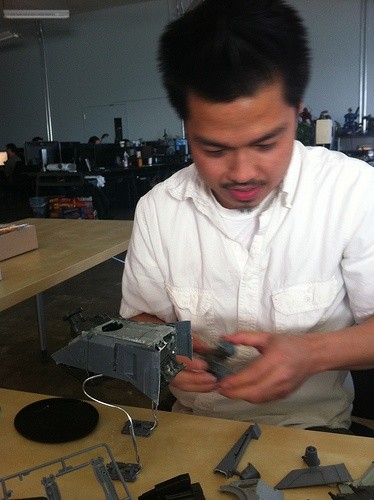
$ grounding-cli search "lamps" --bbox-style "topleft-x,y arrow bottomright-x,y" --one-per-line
3,9 -> 70,19
315,119 -> 333,148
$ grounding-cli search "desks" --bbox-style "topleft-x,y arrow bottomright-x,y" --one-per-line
0,387 -> 373,500
1,218 -> 135,352
1,162 -> 189,214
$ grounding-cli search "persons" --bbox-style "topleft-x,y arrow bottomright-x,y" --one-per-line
32,137 -> 44,142
299,108 -> 312,122
313,110 -> 332,149
0,143 -> 22,183
119,0 -> 374,434
88,136 -> 101,144
344,108 -> 354,122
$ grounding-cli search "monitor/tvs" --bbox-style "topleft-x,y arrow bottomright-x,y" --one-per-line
0,138 -> 190,172
